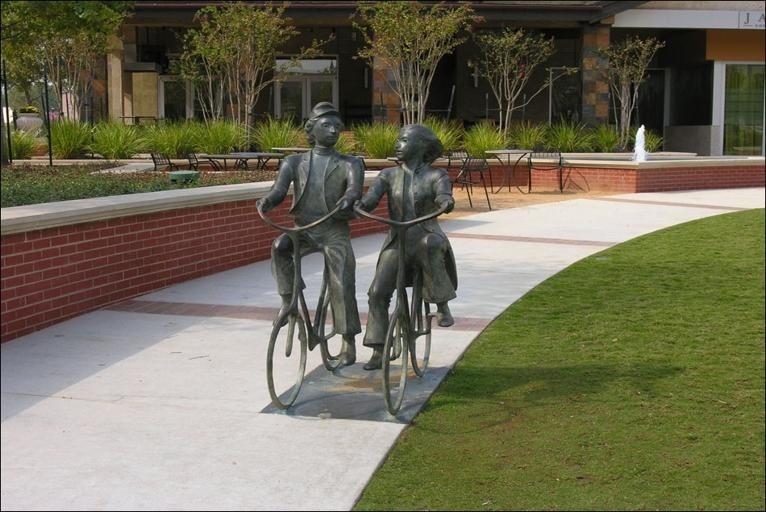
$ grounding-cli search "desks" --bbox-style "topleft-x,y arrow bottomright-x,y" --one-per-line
484,150 -> 534,194
387,156 -> 400,165
198,147 -> 311,171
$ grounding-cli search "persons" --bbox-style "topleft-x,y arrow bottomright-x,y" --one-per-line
255,103 -> 363,365
354,126 -> 459,370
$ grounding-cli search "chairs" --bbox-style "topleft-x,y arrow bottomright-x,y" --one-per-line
151,152 -> 178,172
447,148 -> 493,211
524,148 -> 563,194
186,149 -> 223,172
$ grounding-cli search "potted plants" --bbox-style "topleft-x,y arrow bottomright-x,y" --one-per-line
15,105 -> 43,133
